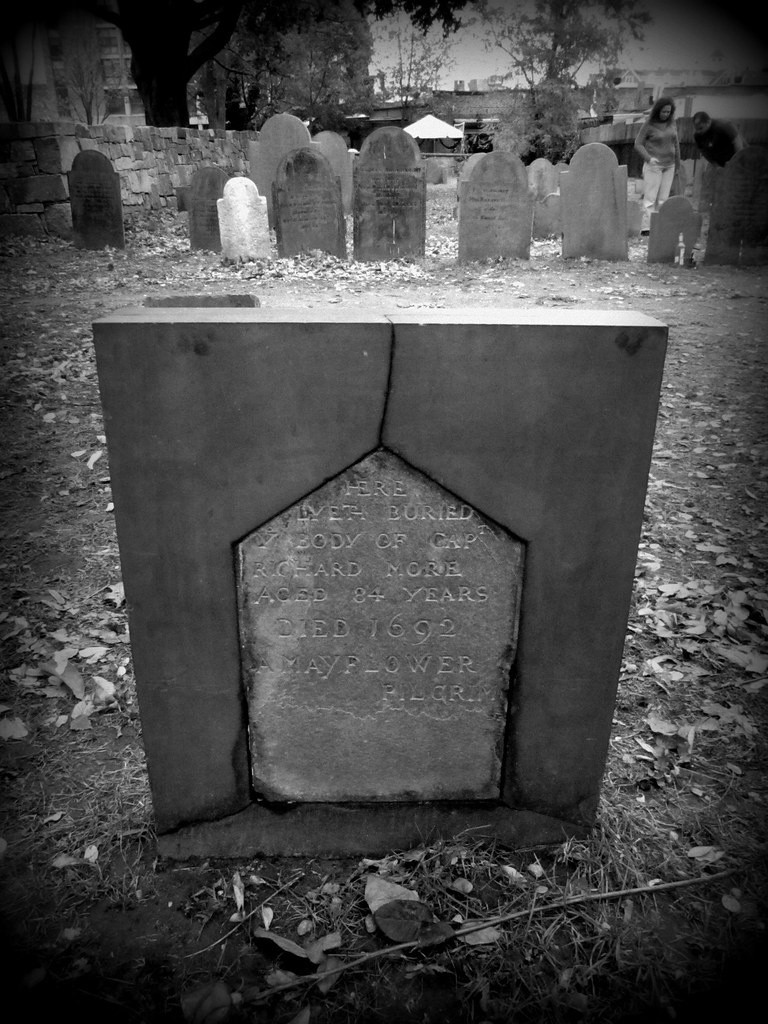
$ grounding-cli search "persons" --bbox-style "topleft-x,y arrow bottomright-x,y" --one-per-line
633,96 -> 681,236
693,110 -> 750,168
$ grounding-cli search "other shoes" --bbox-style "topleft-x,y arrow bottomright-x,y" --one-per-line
641,230 -> 649,237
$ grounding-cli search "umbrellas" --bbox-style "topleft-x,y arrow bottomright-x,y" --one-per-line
400,113 -> 461,154
